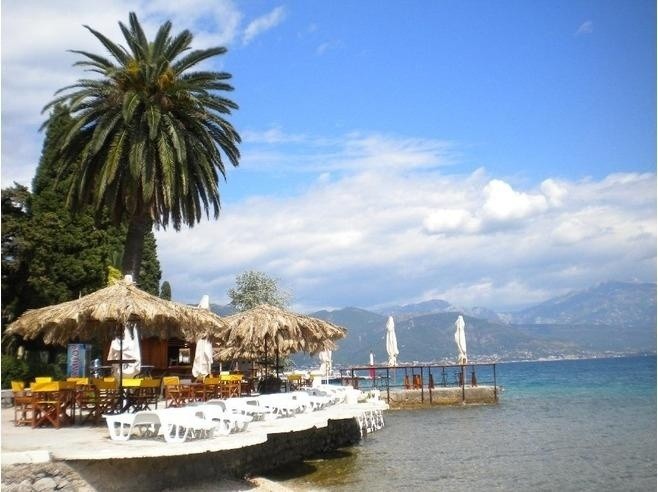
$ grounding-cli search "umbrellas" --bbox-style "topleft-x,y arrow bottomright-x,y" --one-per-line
385,315 -> 400,365
206,302 -> 348,394
5,280 -> 230,414
454,313 -> 469,363
106,272 -> 143,380
368,349 -> 376,378
191,293 -> 214,381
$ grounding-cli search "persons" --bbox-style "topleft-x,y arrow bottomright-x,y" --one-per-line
459,373 -> 464,385
428,374 -> 434,388
404,375 -> 411,388
472,372 -> 477,387
415,375 -> 420,389
